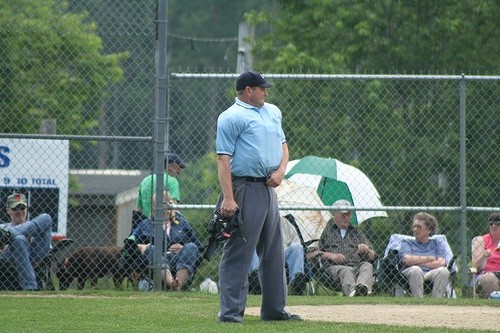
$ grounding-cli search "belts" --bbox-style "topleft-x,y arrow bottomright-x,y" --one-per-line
247,176 -> 267,183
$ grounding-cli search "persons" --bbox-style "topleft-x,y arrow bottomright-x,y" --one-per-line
0,193 -> 53,291
471,212 -> 500,298
315,199 -> 378,297
137,154 -> 186,220
398,212 -> 450,298
133,190 -> 205,293
248,217 -> 305,296
216,72 -> 303,325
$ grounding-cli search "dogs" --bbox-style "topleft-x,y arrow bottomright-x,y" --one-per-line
55,245 -> 123,291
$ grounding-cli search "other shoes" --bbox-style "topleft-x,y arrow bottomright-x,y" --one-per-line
290,272 -> 306,295
348,284 -> 369,296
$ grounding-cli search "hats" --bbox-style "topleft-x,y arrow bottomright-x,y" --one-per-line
164,154 -> 188,168
6,193 -> 28,209
236,71 -> 274,91
331,199 -> 354,214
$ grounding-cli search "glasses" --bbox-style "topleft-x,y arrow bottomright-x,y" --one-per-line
489,221 -> 500,226
10,206 -> 26,210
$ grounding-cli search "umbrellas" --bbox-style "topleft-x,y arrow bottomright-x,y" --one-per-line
272,156 -> 388,243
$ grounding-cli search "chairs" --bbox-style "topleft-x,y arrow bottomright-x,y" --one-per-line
467,236 -> 500,300
0,241 -> 55,290
124,210 -> 187,293
315,240 -> 380,296
384,234 -> 457,298
248,213 -> 315,296
